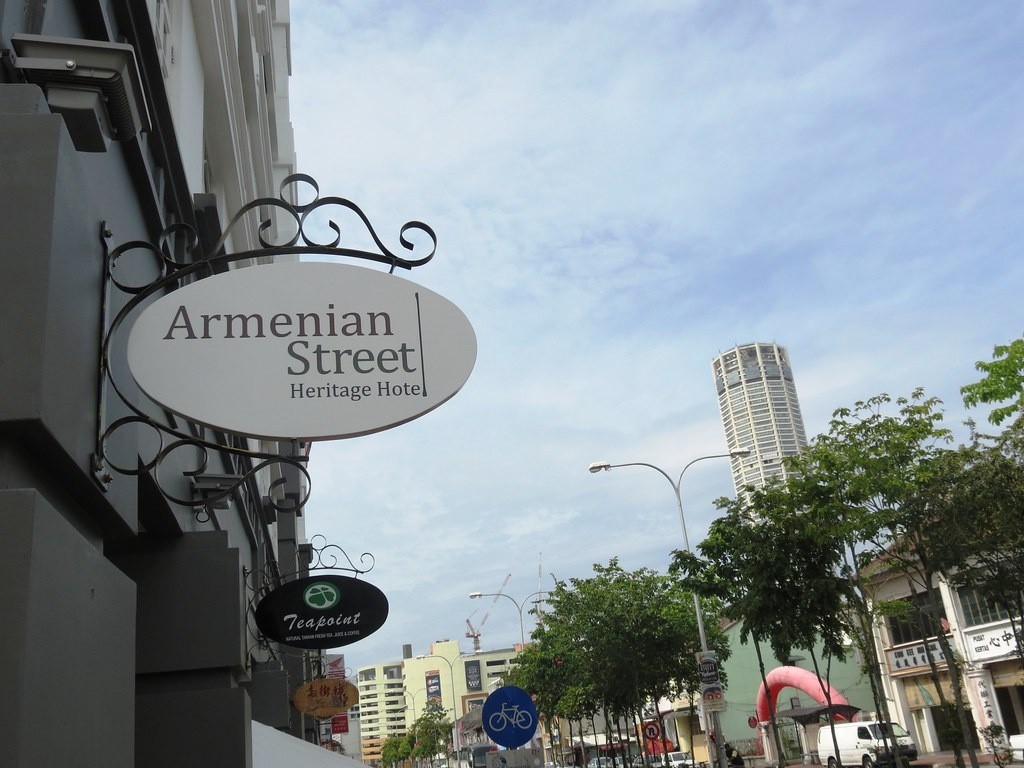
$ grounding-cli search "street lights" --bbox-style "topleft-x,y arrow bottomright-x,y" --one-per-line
469,590 -> 555,655
416,652 -> 466,768
389,687 -> 437,768
588,447 -> 751,768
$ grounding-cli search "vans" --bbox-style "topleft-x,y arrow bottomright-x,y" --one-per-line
816,720 -> 917,768
448,743 -> 498,768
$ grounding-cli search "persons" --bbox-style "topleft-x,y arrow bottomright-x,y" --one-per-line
724,743 -> 746,768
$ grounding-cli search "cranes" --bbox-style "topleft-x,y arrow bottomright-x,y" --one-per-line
464,573 -> 512,655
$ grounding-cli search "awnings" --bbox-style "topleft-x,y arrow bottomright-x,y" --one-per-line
774,704 -> 862,728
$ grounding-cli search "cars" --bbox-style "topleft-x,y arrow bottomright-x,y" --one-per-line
661,751 -> 700,768
587,756 -> 614,768
609,755 -> 636,768
632,754 -> 662,768
